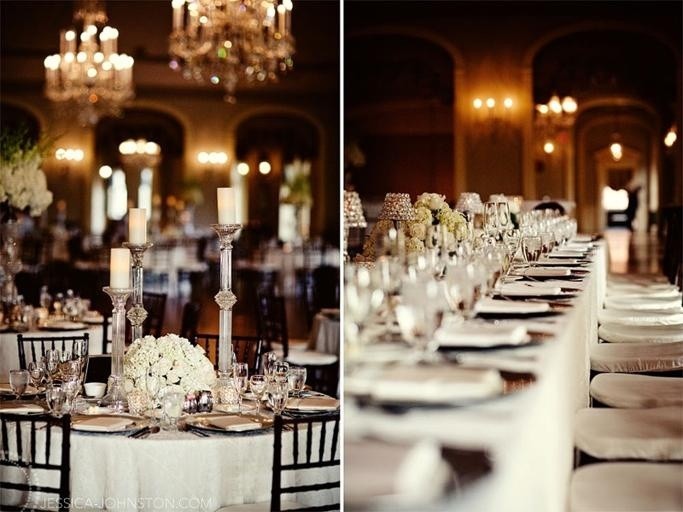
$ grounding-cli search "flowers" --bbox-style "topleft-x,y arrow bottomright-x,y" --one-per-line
409,194 -> 432,238
0,111 -> 63,218
437,202 -> 467,243
416,192 -> 447,211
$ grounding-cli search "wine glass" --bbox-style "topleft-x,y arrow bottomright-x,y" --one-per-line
347,207 -> 580,354
8,339 -> 308,432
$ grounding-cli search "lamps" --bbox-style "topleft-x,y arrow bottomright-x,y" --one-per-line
171,1 -> 293,104
45,0 -> 134,128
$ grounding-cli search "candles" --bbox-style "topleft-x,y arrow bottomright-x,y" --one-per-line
110,248 -> 131,290
128,208 -> 147,246
217,187 -> 235,225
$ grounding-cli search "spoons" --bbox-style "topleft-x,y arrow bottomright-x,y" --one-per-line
135,427 -> 160,439
